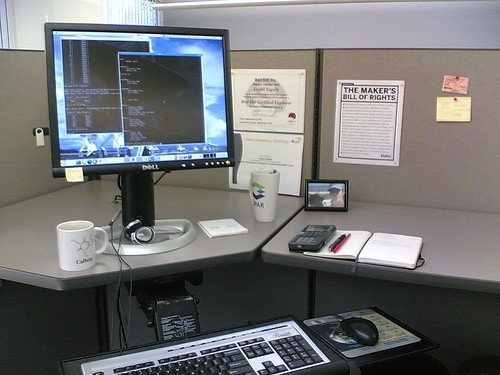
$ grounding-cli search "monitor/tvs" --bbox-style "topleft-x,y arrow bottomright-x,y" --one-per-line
44,23 -> 235,256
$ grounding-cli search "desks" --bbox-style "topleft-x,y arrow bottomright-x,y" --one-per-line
260,196 -> 500,320
1,178 -> 306,352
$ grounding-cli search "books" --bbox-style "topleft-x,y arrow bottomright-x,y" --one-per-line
197,218 -> 249,239
303,230 -> 425,270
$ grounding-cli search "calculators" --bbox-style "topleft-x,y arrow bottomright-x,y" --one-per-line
288,225 -> 336,251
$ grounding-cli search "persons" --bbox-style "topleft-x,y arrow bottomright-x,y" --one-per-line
327,183 -> 345,208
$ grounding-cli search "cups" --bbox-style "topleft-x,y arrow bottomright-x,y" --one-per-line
56,219 -> 109,272
248,169 -> 281,223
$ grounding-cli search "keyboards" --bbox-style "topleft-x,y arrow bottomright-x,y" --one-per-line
58,314 -> 350,374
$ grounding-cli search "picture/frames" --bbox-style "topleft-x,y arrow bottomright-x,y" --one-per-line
305,179 -> 348,211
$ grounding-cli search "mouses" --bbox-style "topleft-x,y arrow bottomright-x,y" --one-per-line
341,317 -> 379,346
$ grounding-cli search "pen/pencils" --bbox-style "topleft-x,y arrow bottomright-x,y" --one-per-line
333,233 -> 351,252
328,234 -> 346,251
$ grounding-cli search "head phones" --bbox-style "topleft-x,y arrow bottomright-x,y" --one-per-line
124,219 -> 181,245
